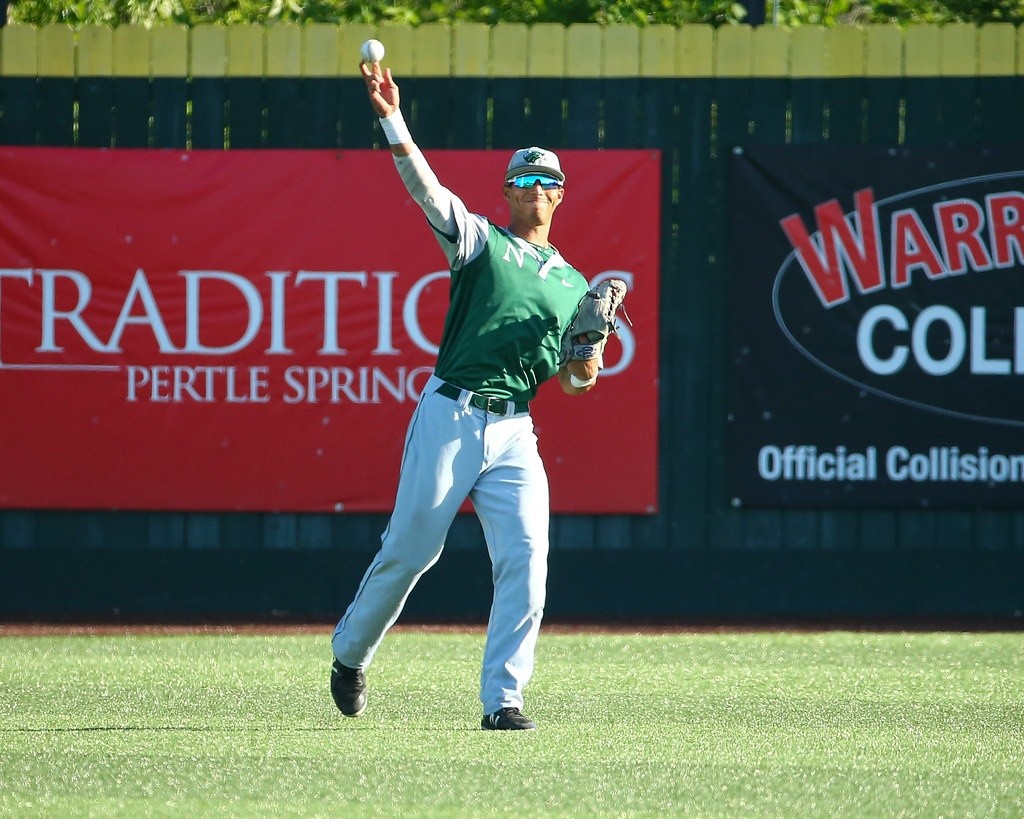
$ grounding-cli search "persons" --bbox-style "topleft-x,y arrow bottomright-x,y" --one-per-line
330,59 -> 628,730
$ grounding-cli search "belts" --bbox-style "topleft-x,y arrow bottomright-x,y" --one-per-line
435,384 -> 530,416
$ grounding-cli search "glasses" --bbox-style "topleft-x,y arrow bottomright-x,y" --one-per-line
507,171 -> 564,188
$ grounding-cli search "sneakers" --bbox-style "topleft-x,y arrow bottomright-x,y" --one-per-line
331,653 -> 367,718
481,704 -> 536,730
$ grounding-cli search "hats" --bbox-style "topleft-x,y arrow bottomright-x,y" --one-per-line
504,147 -> 565,183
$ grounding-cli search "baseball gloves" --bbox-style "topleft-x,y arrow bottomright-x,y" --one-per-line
556,277 -> 629,368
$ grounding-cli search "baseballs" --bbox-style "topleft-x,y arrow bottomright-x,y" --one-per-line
360,39 -> 386,62
